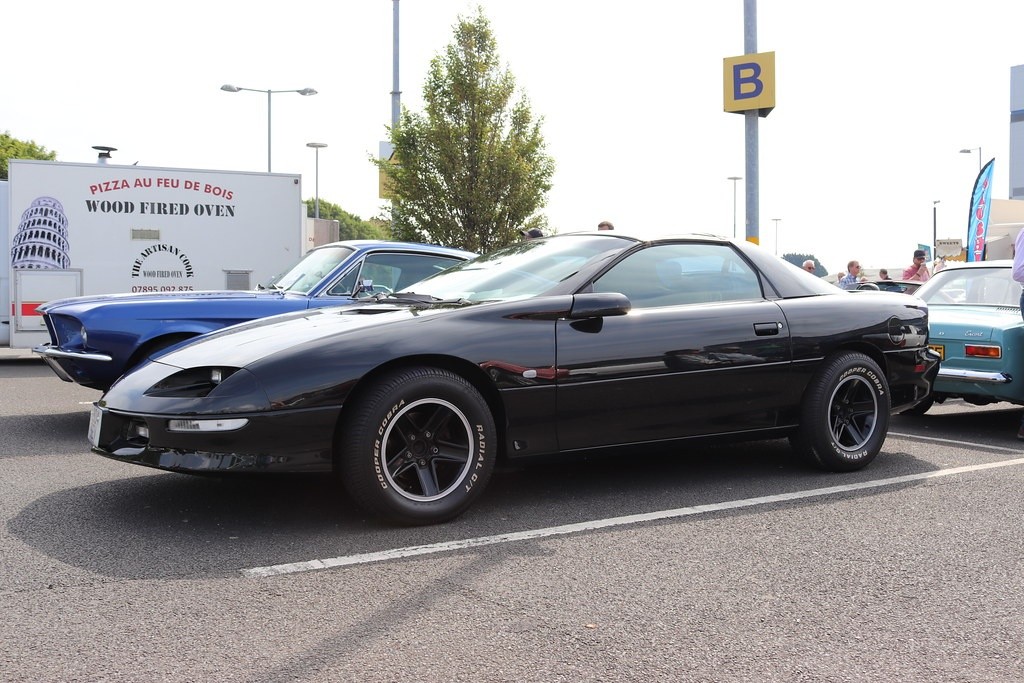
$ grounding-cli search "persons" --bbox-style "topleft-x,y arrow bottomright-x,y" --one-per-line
1012,227 -> 1024,323
833,260 -> 868,290
935,260 -> 945,272
519,228 -> 543,241
879,269 -> 892,280
598,221 -> 614,231
903,249 -> 930,282
802,260 -> 815,274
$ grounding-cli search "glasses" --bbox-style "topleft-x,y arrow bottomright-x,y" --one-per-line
808,267 -> 815,270
856,266 -> 861,269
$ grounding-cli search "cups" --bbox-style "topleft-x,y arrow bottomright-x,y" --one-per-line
921,263 -> 926,267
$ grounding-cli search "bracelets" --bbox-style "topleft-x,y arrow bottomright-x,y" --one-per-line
916,272 -> 920,277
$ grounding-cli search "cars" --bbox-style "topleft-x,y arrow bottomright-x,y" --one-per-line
842,281 -> 966,304
88,230 -> 944,527
899,259 -> 1024,417
32,238 -> 558,394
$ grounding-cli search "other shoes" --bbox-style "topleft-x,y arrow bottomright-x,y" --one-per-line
1017,429 -> 1024,439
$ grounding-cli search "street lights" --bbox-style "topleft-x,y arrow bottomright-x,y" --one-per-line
960,147 -> 981,172
307,143 -> 328,218
728,177 -> 743,238
772,219 -> 782,255
220,84 -> 317,173
933,200 -> 940,267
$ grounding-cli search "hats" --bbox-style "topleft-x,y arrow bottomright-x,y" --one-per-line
914,250 -> 926,258
519,228 -> 543,238
